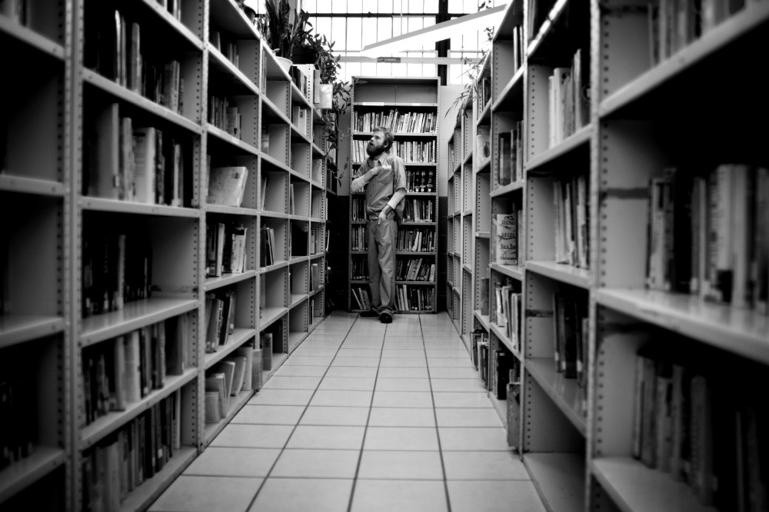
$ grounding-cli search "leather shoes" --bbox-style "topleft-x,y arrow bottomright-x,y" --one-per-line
379,313 -> 392,323
360,310 -> 379,317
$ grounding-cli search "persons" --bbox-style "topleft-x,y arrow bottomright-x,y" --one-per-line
350,125 -> 409,324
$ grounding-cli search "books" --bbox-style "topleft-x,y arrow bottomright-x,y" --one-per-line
350,107 -> 437,312
79,219 -> 320,511
470,0 -> 769,314
2,1 -> 311,218
468,275 -> 769,512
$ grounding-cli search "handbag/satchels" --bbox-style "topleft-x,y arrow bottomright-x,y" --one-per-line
392,161 -> 405,222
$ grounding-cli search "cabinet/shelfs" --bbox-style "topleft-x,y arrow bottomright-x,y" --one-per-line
449,2 -> 768,512
1,2 -> 329,512
351,72 -> 439,313
